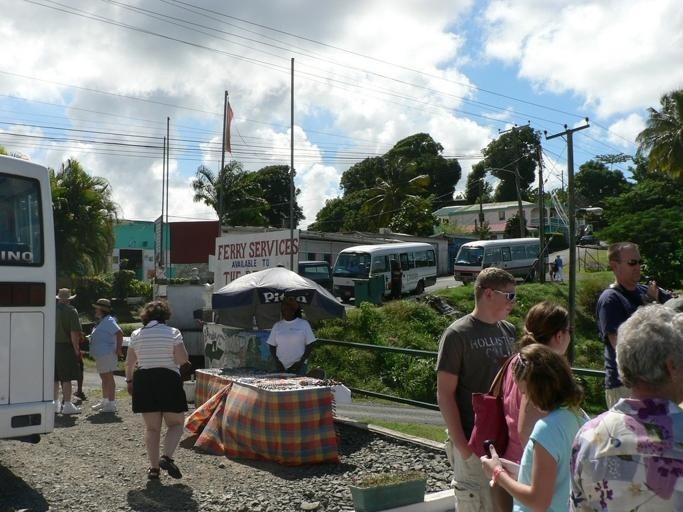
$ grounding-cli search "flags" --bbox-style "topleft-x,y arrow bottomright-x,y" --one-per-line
224,101 -> 233,152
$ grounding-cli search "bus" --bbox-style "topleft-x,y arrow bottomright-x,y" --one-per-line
0,153 -> 58,445
452,236 -> 549,286
331,241 -> 438,303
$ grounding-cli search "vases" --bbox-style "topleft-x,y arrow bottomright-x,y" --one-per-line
183,379 -> 196,401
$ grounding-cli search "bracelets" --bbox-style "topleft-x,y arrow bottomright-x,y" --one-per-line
125,380 -> 133,383
489,467 -> 506,488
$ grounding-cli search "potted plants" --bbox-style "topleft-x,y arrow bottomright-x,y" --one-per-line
345,468 -> 426,512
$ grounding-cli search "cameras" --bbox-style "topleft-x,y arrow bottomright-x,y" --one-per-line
482,439 -> 495,457
655,286 -> 673,304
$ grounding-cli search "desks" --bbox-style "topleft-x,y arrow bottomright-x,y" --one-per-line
191,370 -> 338,466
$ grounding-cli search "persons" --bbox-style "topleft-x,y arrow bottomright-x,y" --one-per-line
434,267 -> 518,512
350,260 -> 359,272
568,304 -> 683,512
532,255 -> 563,282
90,299 -> 123,412
266,299 -> 316,376
61,360 -> 86,404
124,300 -> 188,479
492,302 -> 571,512
54,288 -> 82,415
597,241 -> 671,409
480,343 -> 590,512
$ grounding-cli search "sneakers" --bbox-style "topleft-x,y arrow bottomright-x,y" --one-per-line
75,392 -> 86,399
53,400 -> 61,414
62,403 -> 81,414
92,399 -> 116,413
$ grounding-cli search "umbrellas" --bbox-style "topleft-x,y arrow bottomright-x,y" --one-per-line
212,266 -> 347,330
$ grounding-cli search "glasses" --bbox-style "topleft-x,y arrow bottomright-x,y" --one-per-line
620,259 -> 644,265
492,289 -> 515,300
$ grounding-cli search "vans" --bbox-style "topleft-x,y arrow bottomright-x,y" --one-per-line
299,260 -> 332,298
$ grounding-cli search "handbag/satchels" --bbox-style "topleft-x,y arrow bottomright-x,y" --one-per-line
468,392 -> 507,457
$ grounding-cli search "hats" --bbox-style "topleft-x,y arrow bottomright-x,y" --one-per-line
92,299 -> 112,313
56,288 -> 77,300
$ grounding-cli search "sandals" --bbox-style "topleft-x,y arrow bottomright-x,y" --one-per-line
148,468 -> 159,479
159,455 -> 182,479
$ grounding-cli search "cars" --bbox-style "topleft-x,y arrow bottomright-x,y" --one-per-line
579,235 -> 600,246
